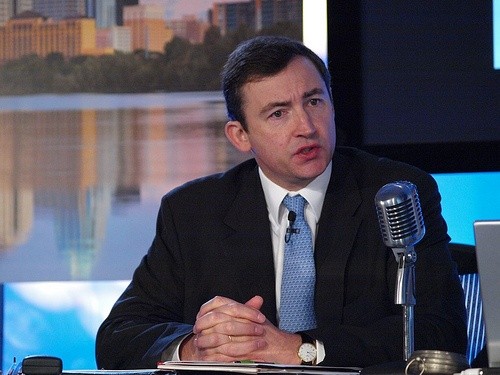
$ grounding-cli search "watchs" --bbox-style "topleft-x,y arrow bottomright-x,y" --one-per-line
294,331 -> 317,366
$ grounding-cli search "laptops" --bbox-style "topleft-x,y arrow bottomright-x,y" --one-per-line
473,221 -> 500,367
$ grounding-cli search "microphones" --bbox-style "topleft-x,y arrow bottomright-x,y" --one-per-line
374,181 -> 426,363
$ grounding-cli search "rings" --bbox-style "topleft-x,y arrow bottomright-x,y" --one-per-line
228,336 -> 232,342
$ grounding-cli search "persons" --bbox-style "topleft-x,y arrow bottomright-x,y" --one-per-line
95,35 -> 467,375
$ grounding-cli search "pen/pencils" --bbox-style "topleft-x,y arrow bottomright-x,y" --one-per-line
234,360 -> 275,365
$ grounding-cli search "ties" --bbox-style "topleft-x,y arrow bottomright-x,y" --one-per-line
280,194 -> 317,334
